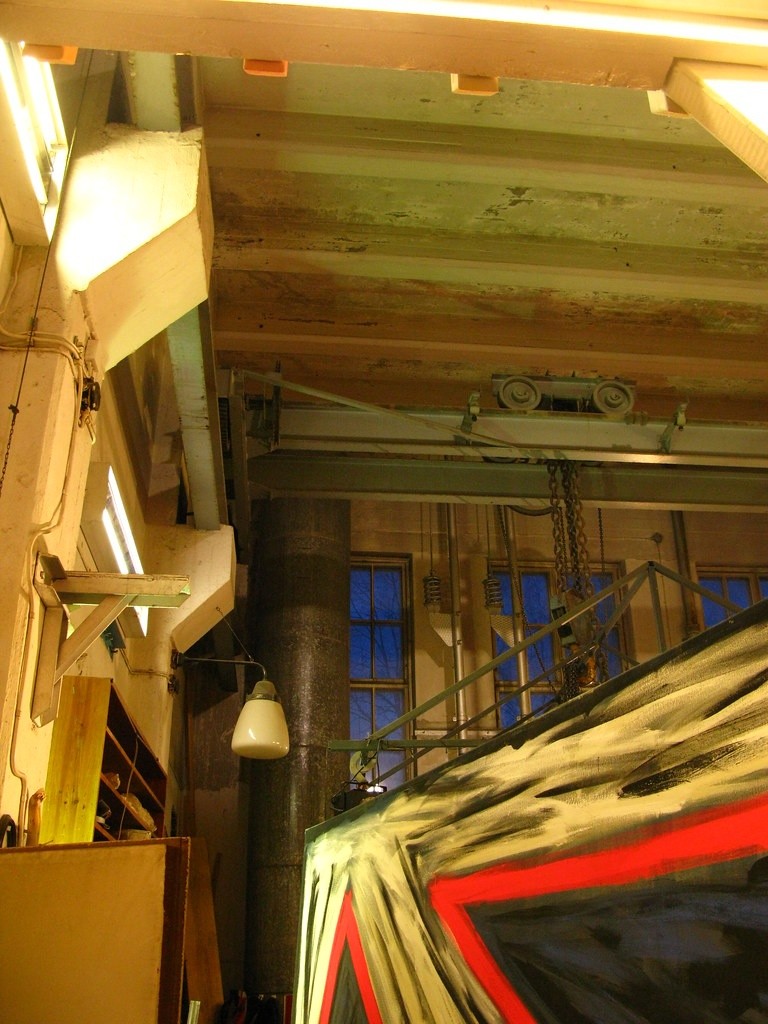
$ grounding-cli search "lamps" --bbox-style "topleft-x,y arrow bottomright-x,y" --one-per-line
170,606 -> 289,760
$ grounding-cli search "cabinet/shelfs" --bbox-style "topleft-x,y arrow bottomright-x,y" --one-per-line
38,673 -> 169,845
